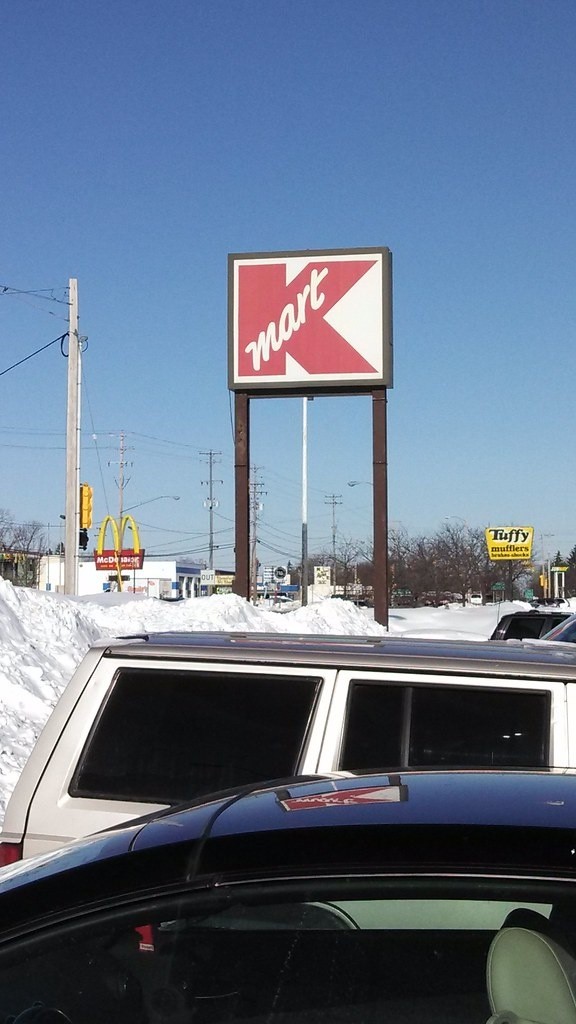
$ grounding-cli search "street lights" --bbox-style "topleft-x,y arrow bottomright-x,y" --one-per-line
116,495 -> 181,592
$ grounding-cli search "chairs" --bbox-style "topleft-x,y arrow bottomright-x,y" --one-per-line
484,908 -> 576,1024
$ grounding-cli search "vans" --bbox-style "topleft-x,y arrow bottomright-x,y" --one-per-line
471,593 -> 484,605
1,631 -> 576,871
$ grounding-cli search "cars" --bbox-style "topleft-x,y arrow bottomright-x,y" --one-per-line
489,609 -> 576,643
0,767 -> 576,1024
